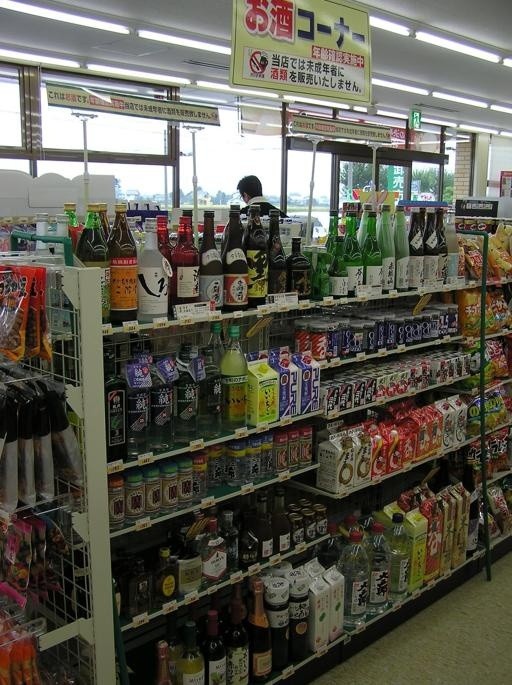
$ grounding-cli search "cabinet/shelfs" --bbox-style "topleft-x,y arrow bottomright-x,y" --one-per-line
7,218 -> 511,685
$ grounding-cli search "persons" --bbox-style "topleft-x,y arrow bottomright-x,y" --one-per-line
237,175 -> 291,218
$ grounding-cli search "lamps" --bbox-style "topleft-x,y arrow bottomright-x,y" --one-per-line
0,0 -> 512,142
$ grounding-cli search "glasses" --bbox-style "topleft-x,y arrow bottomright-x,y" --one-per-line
238,195 -> 244,201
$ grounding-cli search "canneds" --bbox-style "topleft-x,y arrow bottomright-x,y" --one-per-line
293,303 -> 459,361
287,498 -> 328,546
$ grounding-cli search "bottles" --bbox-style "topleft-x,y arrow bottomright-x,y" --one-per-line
0,203 -> 460,327
292,303 -> 458,362
111,488 -> 411,685
435,455 -> 480,559
62,320 -> 312,532
